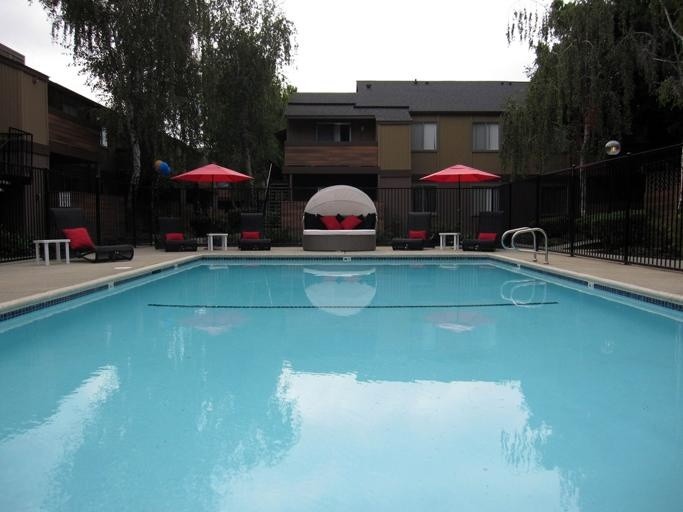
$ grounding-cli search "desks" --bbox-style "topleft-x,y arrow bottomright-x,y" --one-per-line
33,239 -> 71,265
439,232 -> 462,252
206,233 -> 229,251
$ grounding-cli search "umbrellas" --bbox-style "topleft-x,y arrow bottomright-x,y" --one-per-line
155,162 -> 256,228
418,163 -> 502,233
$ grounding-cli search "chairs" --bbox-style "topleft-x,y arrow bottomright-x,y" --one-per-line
40,207 -> 134,263
155,216 -> 197,251
391,212 -> 436,251
462,211 -> 504,252
238,213 -> 271,250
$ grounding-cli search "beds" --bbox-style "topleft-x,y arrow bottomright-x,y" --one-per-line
302,185 -> 379,251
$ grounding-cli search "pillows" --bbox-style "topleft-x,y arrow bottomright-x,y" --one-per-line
162,232 -> 185,242
408,230 -> 427,241
62,226 -> 97,251
478,232 -> 497,241
241,230 -> 261,239
305,211 -> 375,230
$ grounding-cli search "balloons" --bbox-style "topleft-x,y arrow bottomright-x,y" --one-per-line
153,159 -> 171,176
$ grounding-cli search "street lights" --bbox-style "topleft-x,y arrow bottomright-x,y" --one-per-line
95,117 -> 111,246
